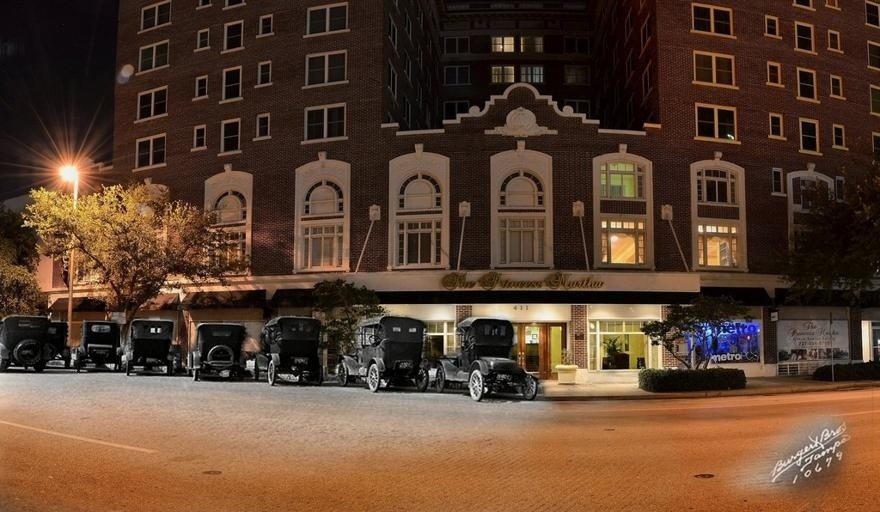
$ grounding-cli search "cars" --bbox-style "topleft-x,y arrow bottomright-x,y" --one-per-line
435,317 -> 539,401
0,315 -> 73,374
125,318 -> 175,376
337,315 -> 430,393
254,316 -> 322,387
190,322 -> 248,381
74,319 -> 123,373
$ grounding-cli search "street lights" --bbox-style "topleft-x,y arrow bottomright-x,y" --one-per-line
59,165 -> 79,349
